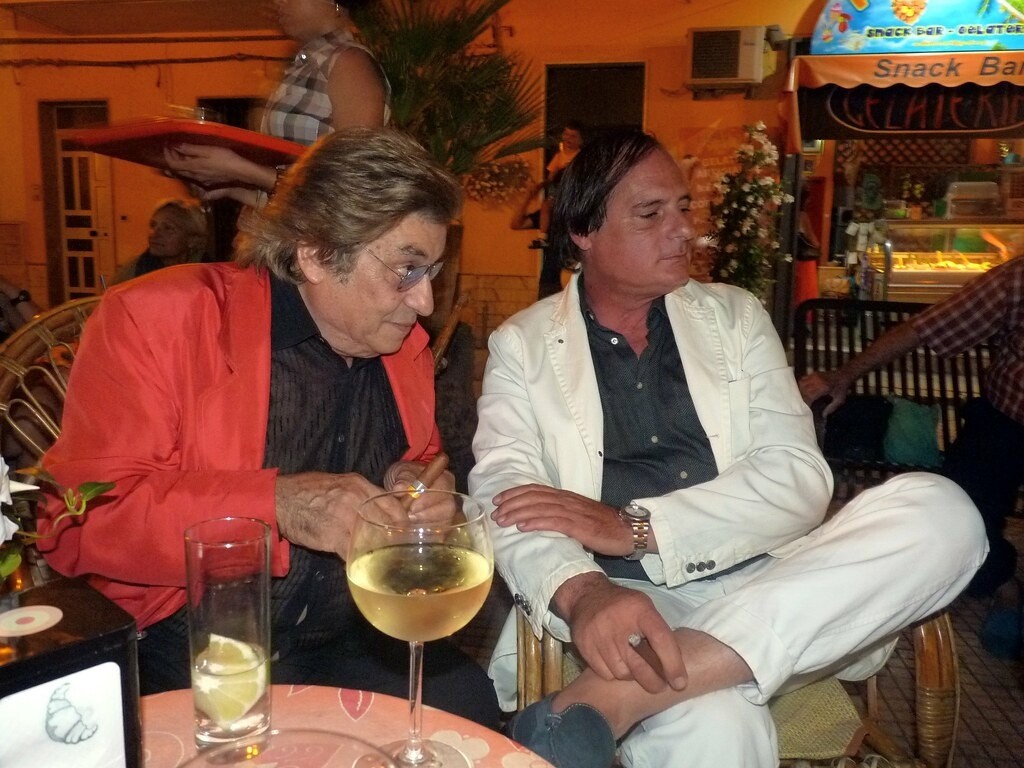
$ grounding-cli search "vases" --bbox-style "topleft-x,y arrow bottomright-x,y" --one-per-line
751,286 -> 776,321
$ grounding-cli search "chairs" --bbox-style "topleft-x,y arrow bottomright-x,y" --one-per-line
513,594 -> 961,768
0,297 -> 98,586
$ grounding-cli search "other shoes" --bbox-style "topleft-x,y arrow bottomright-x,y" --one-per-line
982,577 -> 1024,657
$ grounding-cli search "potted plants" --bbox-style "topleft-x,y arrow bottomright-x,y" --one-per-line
336,0 -> 561,348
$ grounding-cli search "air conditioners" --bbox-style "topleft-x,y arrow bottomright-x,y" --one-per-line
686,26 -> 764,88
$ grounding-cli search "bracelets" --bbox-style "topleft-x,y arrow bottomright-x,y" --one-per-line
254,189 -> 261,210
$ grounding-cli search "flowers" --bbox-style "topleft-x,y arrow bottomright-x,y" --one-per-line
696,119 -> 795,305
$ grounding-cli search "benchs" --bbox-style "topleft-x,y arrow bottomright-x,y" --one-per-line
791,300 -> 1024,520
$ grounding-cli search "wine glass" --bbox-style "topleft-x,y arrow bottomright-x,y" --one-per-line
345,489 -> 493,768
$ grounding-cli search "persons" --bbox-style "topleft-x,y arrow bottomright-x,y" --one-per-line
510,125 -> 591,296
797,251 -> 1024,607
163,0 -> 394,212
463,127 -> 990,768
0,126 -> 504,731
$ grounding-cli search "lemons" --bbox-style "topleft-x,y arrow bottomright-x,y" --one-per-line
207,632 -> 265,729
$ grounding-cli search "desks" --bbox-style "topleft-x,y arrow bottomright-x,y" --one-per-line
135,684 -> 557,768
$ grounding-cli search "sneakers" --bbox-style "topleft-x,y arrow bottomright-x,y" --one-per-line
509,691 -> 616,768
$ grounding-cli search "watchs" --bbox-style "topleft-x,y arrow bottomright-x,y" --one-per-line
618,504 -> 651,561
275,163 -> 286,181
10,290 -> 32,307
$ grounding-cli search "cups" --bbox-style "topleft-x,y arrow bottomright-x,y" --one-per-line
183,515 -> 272,763
170,106 -> 225,124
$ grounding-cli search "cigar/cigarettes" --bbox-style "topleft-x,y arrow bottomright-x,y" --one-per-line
401,452 -> 450,512
626,631 -> 670,687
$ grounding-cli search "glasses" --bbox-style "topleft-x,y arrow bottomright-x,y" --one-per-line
348,237 -> 444,292
